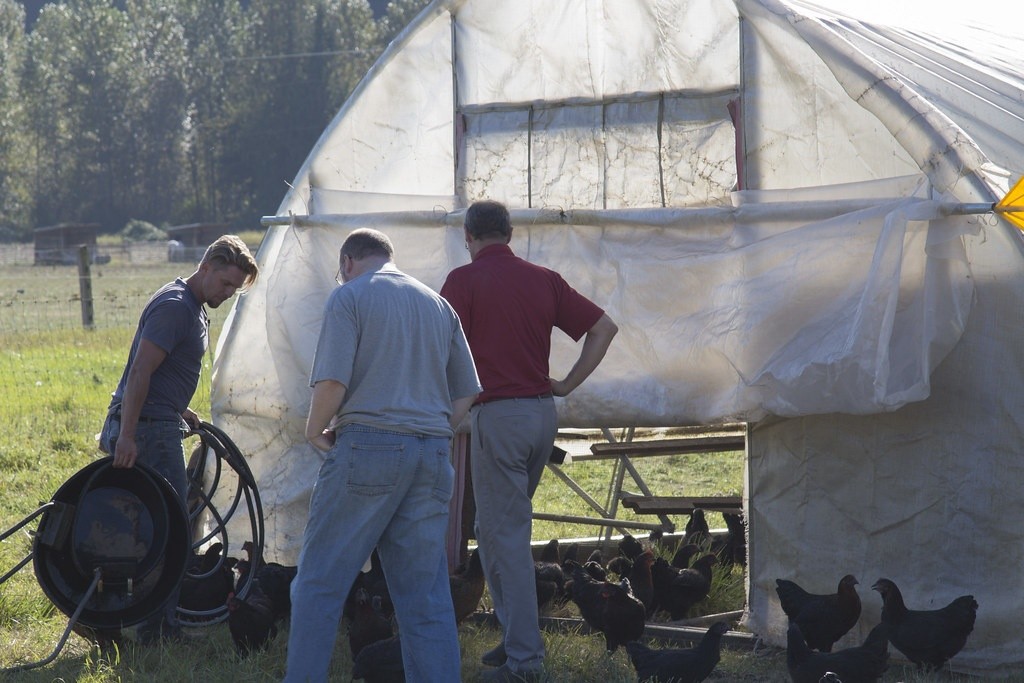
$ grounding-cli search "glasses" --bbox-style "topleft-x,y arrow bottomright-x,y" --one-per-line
335,259 -> 345,286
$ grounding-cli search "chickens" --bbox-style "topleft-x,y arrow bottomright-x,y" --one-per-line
775,574 -> 979,683
449,546 -> 485,628
534,507 -> 746,683
343,550 -> 407,682
181,540 -> 297,664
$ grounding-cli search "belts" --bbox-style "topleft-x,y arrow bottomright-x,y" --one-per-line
471,392 -> 555,407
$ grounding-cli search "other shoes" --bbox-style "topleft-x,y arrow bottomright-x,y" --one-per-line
480,663 -> 543,683
481,642 -> 507,666
137,629 -> 208,647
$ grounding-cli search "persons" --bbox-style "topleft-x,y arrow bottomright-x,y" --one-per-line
286,228 -> 483,683
439,200 -> 619,681
98,235 -> 258,649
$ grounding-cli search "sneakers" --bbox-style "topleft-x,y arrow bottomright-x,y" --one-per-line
69,619 -> 138,653
